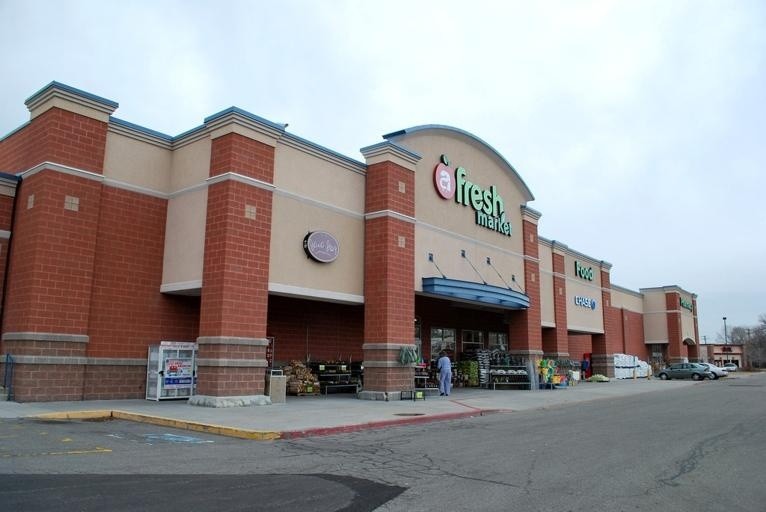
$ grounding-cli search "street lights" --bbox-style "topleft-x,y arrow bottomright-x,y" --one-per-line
721,316 -> 728,363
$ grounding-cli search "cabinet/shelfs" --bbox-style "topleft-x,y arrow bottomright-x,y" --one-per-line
145,340 -> 199,402
263,336 -> 360,406
415,347 -> 529,395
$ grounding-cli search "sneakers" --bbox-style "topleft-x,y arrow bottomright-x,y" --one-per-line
440,393 -> 448,396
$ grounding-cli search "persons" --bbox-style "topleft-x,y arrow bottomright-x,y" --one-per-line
436,351 -> 452,396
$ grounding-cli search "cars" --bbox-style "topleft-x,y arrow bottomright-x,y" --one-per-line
651,362 -> 710,382
718,363 -> 739,372
697,362 -> 728,380
